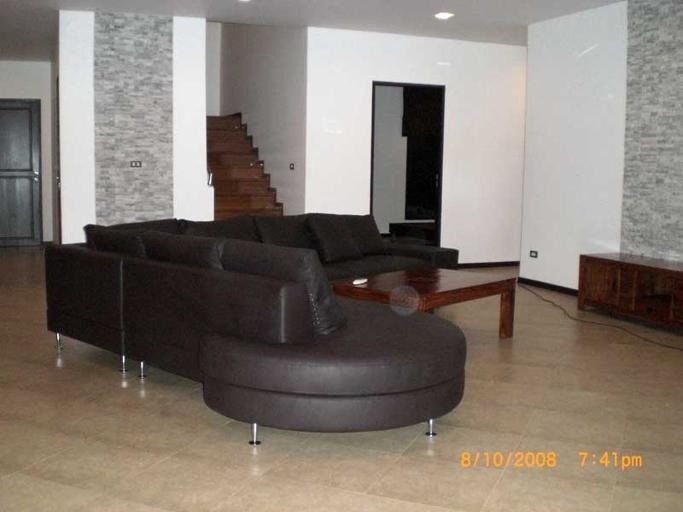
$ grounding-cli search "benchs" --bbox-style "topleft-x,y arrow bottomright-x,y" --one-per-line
42,213 -> 467,444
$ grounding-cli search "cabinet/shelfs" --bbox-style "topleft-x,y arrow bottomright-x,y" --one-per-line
578,251 -> 683,335
